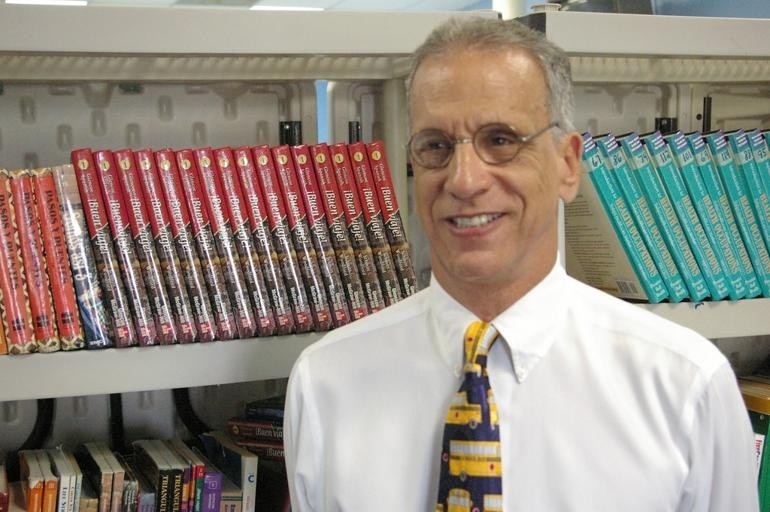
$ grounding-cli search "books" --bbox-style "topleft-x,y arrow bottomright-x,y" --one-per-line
1,395 -> 291,512
565,127 -> 770,304
2,142 -> 417,354
737,374 -> 770,512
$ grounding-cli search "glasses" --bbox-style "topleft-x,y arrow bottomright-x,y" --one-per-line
403,122 -> 560,169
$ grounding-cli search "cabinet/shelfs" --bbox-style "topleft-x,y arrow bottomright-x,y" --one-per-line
508,3 -> 770,378
1,4 -> 502,402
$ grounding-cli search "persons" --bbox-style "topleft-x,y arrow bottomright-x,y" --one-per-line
283,14 -> 762,511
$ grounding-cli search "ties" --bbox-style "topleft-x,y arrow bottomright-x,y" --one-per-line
435,321 -> 502,511
